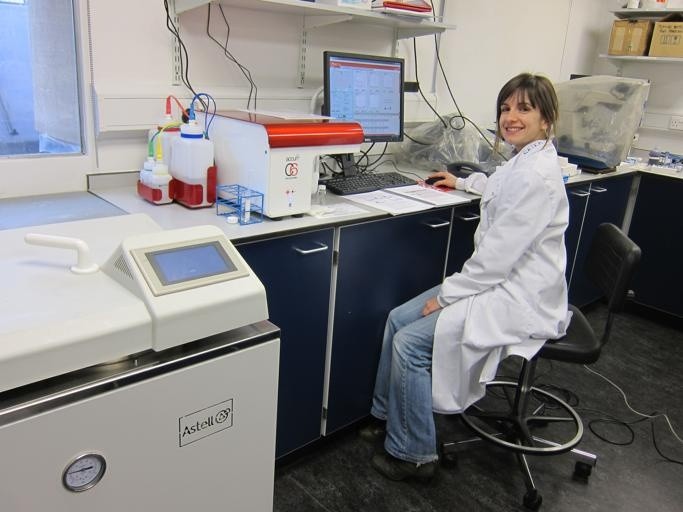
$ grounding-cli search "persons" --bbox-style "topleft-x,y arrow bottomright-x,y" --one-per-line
359,72 -> 574,480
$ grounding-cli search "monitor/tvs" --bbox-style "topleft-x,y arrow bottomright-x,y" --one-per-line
323,50 -> 405,178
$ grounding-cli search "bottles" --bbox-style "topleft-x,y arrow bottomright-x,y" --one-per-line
237,188 -> 251,224
316,184 -> 327,207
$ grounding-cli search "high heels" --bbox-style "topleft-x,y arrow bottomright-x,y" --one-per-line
372,455 -> 439,485
358,427 -> 386,440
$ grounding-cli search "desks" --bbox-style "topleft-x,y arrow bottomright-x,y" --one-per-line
98,159 -> 683,468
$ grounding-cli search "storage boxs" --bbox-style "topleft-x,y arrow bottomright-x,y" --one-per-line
608,13 -> 683,58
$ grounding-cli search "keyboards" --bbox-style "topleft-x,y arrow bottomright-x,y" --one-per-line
325,172 -> 418,195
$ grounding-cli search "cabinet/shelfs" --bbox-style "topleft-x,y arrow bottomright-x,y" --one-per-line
597,7 -> 683,62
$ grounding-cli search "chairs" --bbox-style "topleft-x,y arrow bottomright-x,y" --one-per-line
435,219 -> 645,510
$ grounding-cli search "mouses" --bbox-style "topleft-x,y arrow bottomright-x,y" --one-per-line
425,177 -> 445,185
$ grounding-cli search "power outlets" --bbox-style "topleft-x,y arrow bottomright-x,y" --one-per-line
670,115 -> 683,131
